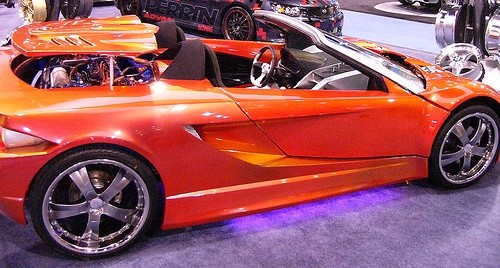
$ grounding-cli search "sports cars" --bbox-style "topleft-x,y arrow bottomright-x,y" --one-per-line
1,10 -> 499,259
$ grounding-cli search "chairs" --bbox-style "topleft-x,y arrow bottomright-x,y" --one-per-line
204,44 -> 226,88
176,24 -> 186,41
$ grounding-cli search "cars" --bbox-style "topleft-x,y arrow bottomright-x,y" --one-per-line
113,0 -> 344,40
